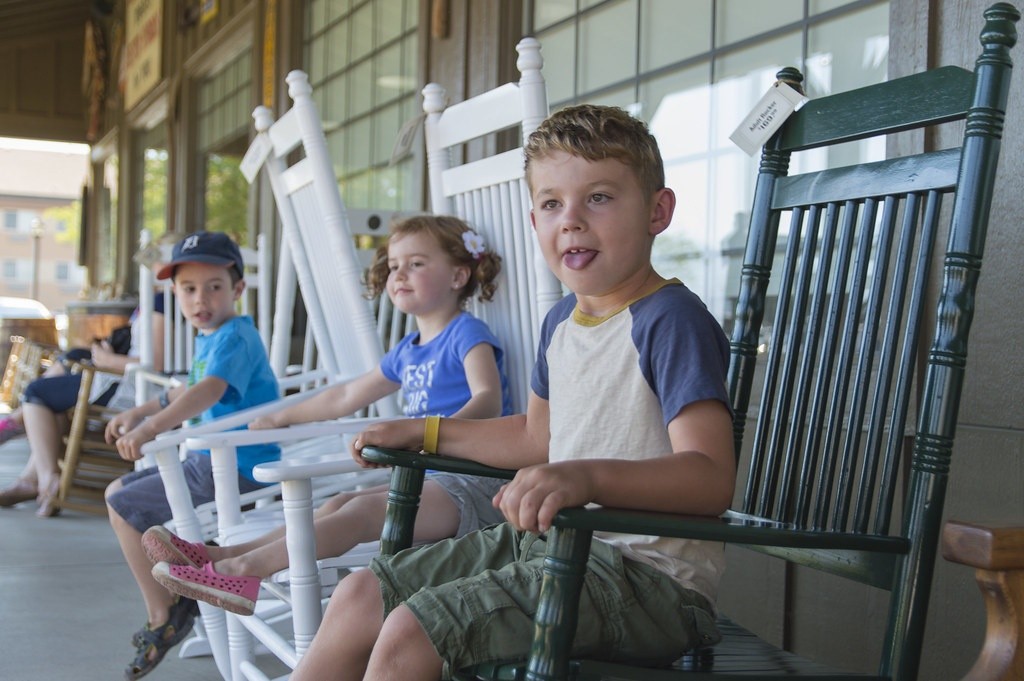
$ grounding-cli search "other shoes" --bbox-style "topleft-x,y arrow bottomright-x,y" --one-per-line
151,560 -> 261,616
0,476 -> 38,507
139,525 -> 214,570
34,471 -> 62,517
0,410 -> 25,440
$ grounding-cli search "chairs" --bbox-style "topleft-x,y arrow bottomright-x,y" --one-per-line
53,0 -> 1024,681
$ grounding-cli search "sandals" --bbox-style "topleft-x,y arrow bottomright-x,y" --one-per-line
132,596 -> 200,649
130,604 -> 195,679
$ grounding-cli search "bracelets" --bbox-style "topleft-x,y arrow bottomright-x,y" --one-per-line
423,415 -> 440,455
159,392 -> 170,408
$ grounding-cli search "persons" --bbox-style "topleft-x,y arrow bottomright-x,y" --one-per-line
286,104 -> 736,681
0,241 -> 185,518
103,229 -> 283,681
141,210 -> 515,617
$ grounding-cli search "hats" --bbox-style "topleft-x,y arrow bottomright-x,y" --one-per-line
156,230 -> 244,280
